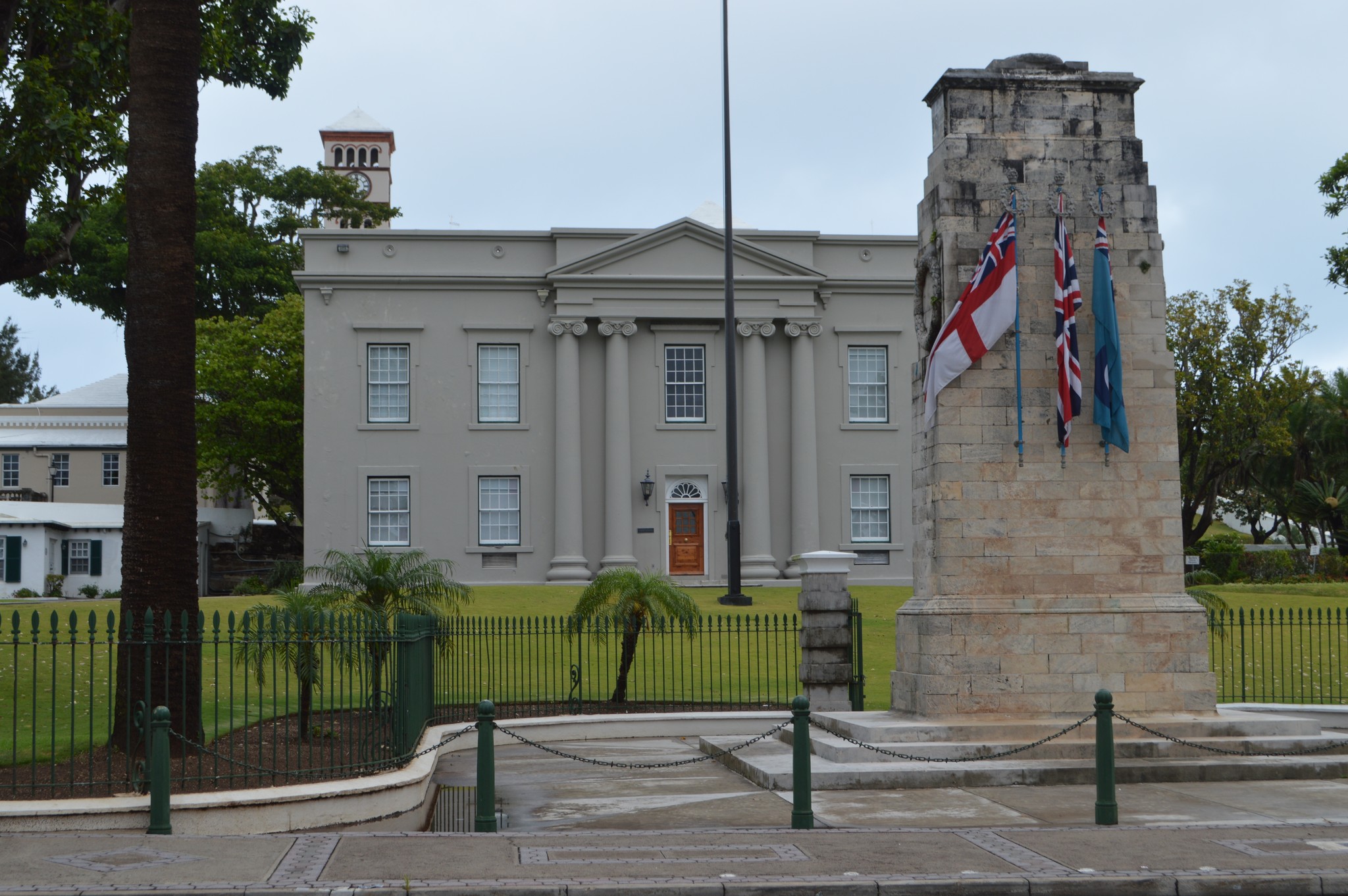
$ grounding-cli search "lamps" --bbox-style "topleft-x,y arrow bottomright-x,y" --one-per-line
639,468 -> 655,506
23,539 -> 27,547
48,458 -> 58,501
721,475 -> 728,505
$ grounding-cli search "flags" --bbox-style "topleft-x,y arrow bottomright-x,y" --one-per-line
1053,188 -> 1082,447
1091,188 -> 1129,454
923,191 -> 1017,423
